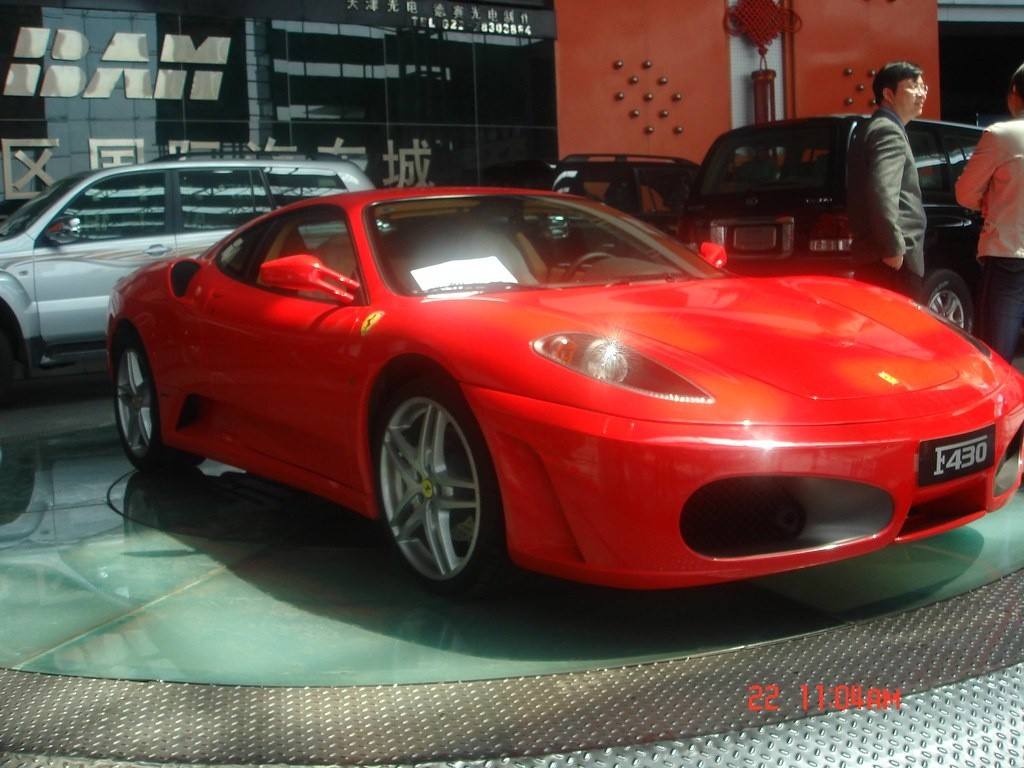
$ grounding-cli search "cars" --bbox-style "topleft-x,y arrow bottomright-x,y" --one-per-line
105,185 -> 1024,606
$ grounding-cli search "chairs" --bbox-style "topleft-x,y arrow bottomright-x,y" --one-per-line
736,157 -> 777,191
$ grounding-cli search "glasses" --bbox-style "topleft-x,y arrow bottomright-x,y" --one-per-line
896,82 -> 928,97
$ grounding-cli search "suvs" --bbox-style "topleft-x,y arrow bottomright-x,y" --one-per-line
0,152 -> 376,408
487,153 -> 703,239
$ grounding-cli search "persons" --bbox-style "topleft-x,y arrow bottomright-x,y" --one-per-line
955,62 -> 1024,365
844,62 -> 928,302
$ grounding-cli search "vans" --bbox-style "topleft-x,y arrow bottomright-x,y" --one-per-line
678,115 -> 988,350
